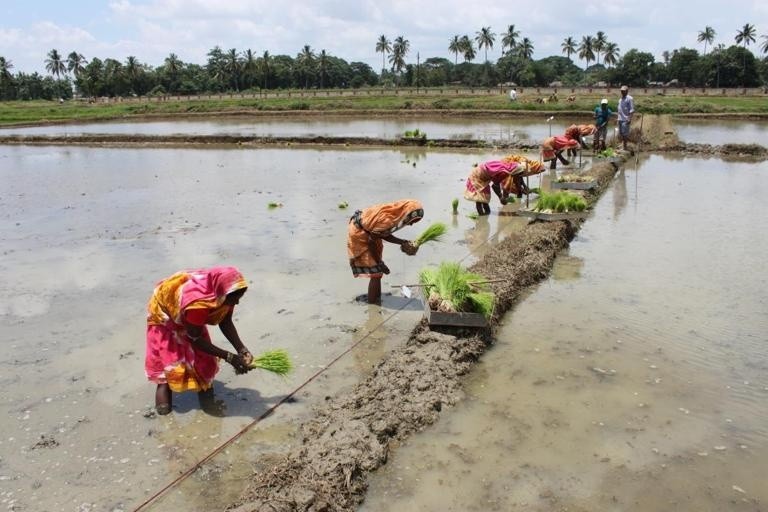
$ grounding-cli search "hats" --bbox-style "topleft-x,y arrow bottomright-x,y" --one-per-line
620,86 -> 628,90
601,99 -> 608,104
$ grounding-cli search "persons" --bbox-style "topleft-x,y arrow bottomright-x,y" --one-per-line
591,98 -> 613,156
562,124 -> 599,158
541,135 -> 582,170
463,160 -> 525,217
614,86 -> 635,152
534,92 -> 577,105
346,199 -> 425,302
510,88 -> 517,103
143,264 -> 257,416
497,155 -> 546,199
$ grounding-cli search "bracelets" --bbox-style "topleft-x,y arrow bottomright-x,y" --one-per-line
225,351 -> 234,364
238,347 -> 250,354
